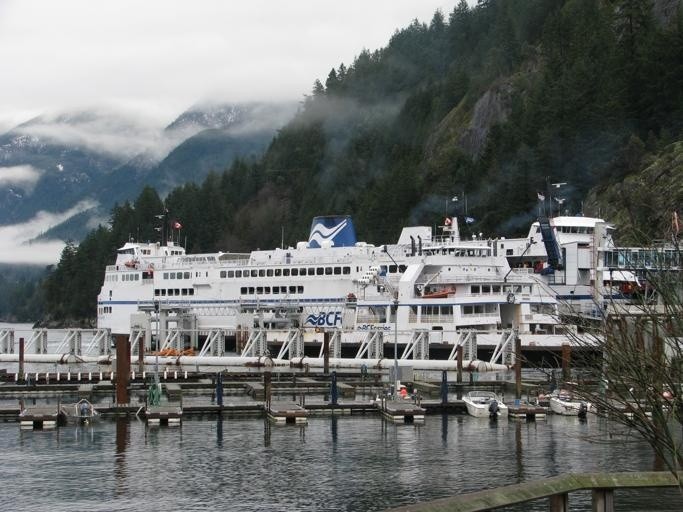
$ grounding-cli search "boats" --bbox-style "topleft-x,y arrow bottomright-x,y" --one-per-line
59,397 -> 101,425
94,178 -> 616,360
461,390 -> 509,418
547,389 -> 593,415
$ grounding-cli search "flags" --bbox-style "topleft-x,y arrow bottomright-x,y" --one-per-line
443,217 -> 451,226
172,220 -> 183,230
464,216 -> 475,224
